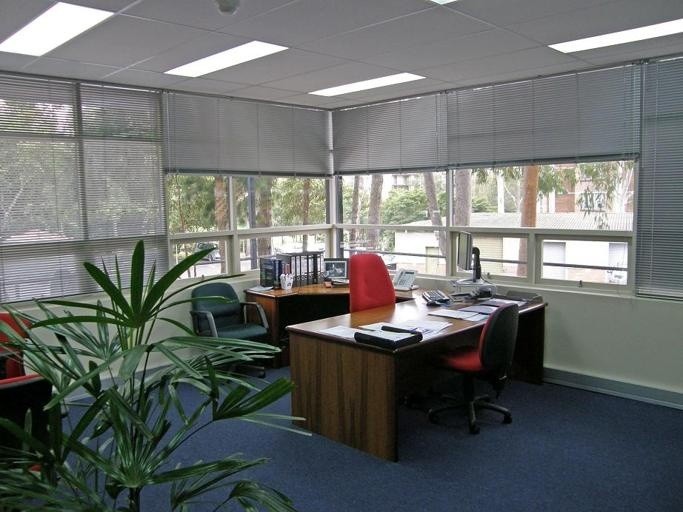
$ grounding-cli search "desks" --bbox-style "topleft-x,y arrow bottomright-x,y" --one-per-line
244,282 -> 450,365
285,297 -> 548,461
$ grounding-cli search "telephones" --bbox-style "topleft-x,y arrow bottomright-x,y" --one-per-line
394,268 -> 417,291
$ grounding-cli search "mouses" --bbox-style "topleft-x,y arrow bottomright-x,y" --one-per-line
426,300 -> 441,306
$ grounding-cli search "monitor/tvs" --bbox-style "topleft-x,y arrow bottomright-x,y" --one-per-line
457,231 -> 473,271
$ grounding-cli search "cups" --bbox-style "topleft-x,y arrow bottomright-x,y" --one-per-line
280,274 -> 293,289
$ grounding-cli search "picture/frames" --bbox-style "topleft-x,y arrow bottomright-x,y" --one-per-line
324,258 -> 348,281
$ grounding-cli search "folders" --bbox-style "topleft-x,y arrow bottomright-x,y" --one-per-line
277,252 -> 325,287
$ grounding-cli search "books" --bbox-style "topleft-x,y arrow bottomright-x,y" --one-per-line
260,255 -> 290,290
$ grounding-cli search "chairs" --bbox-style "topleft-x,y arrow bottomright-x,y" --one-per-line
426,305 -> 519,435
347,254 -> 395,313
189,282 -> 270,383
0,374 -> 52,472
0,313 -> 32,378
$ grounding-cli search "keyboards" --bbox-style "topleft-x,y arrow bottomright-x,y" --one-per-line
422,290 -> 450,302
